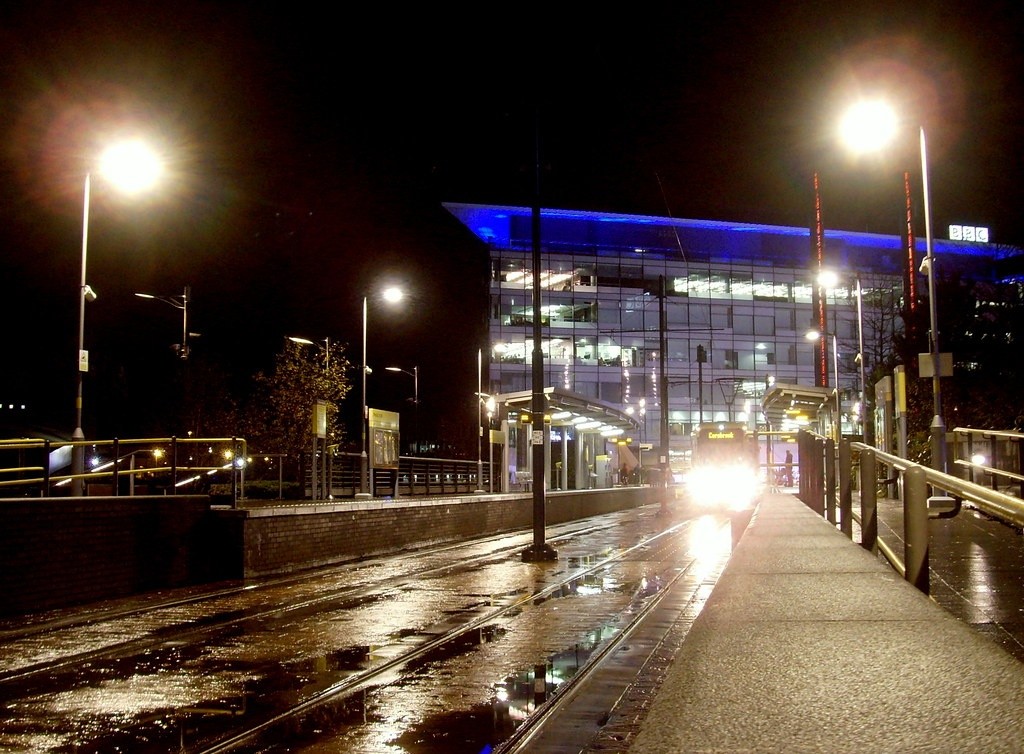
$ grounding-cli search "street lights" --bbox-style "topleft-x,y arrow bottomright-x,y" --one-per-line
385,364 -> 421,456
819,271 -> 867,441
356,287 -> 403,498
288,336 -> 329,500
487,397 -> 496,493
806,330 -> 841,439
841,104 -> 950,504
71,142 -> 157,497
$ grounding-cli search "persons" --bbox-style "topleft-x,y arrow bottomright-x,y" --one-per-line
785,450 -> 793,487
622,463 -> 627,475
634,464 -> 639,476
